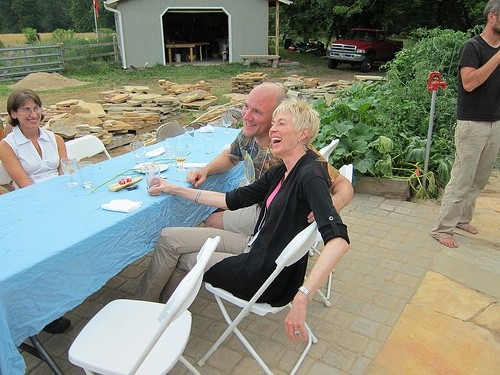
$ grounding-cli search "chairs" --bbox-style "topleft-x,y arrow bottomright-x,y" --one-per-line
156,121 -> 184,141
69,235 -> 222,375
308,139 -> 340,257
64,134 -> 112,162
304,163 -> 353,308
197,220 -> 317,375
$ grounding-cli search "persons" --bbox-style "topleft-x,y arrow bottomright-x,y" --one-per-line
136,97 -> 350,342
432,0 -> 500,247
187,80 -> 354,234
0,87 -> 71,332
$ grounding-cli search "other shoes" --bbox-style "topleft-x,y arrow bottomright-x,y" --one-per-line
44,316 -> 71,333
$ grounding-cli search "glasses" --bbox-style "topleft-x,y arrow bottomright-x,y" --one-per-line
16,107 -> 41,114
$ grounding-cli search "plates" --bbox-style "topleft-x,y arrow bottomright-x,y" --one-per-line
134,163 -> 168,173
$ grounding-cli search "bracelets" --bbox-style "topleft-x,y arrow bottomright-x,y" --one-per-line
195,189 -> 202,203
298,286 -> 313,301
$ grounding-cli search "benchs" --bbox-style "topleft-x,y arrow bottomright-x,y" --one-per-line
240,55 -> 282,68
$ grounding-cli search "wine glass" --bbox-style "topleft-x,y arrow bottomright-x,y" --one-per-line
221,113 -> 232,135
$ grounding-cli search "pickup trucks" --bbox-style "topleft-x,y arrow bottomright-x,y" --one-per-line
325,28 -> 404,73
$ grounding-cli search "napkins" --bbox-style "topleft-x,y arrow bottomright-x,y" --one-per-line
102,198 -> 141,213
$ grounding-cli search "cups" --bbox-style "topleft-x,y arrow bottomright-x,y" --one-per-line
203,135 -> 216,156
165,126 -> 195,173
145,166 -> 160,196
61,158 -> 94,188
132,140 -> 145,164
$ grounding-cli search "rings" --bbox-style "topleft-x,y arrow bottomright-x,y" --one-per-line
295,330 -> 300,336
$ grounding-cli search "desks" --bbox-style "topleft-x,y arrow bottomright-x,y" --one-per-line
0,127 -> 253,375
166,42 -> 210,64
